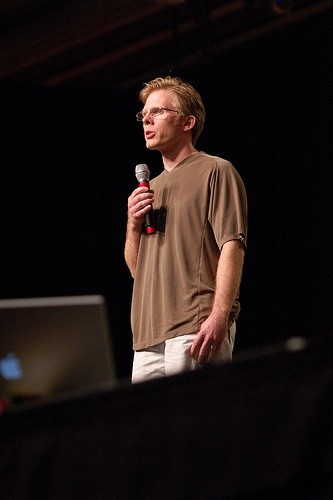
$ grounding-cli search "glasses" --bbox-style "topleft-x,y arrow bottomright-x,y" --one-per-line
136,107 -> 179,121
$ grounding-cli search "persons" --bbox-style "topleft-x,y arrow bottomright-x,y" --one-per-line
124,78 -> 248,383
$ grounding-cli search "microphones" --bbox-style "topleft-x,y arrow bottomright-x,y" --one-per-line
135,164 -> 156,235
